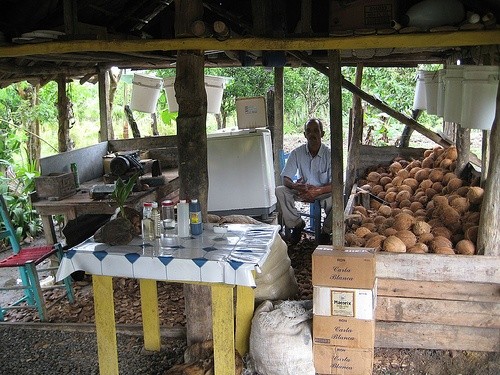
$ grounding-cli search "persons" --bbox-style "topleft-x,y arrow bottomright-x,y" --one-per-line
275,118 -> 341,247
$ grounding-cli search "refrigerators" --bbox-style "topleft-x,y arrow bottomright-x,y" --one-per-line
206,128 -> 278,219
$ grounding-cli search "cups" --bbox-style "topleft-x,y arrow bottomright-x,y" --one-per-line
141,219 -> 156,241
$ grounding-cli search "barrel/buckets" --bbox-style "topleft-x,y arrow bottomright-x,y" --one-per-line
163,77 -> 179,113
204,74 -> 225,114
412,64 -> 500,131
130,73 -> 163,113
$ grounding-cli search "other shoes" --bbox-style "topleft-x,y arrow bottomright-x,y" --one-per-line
318,231 -> 330,245
291,220 -> 306,245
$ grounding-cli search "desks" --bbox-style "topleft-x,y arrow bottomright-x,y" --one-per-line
34,168 -> 179,275
56,223 -> 281,375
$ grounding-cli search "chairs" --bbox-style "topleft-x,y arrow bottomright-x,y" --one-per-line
0,193 -> 75,321
280,150 -> 314,231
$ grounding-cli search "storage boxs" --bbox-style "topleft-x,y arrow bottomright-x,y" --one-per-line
35,172 -> 78,197
312,245 -> 378,375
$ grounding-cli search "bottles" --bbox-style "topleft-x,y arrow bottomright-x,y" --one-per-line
143,203 -> 151,219
177,200 -> 190,237
161,200 -> 175,228
150,202 -> 161,238
71,163 -> 79,188
190,199 -> 202,236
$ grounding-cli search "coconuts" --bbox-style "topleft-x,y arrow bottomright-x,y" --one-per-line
344,146 -> 484,256
